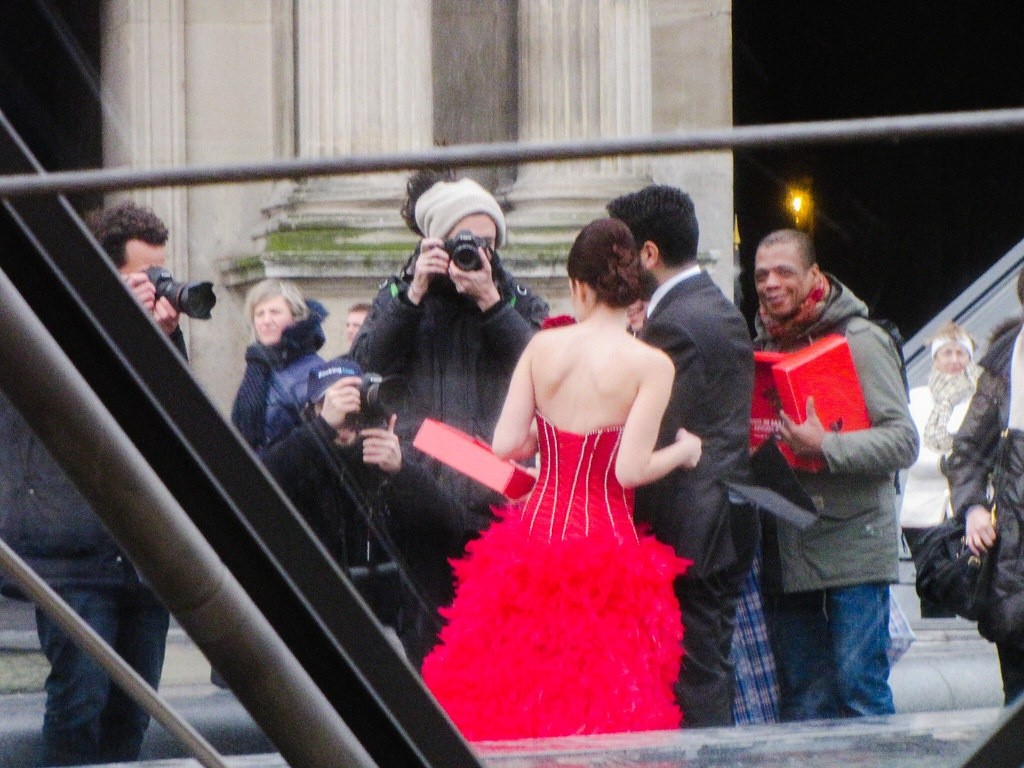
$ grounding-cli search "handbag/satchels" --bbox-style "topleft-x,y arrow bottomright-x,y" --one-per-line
912,514 -> 988,619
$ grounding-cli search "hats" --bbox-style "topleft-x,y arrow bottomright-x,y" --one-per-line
307,360 -> 367,403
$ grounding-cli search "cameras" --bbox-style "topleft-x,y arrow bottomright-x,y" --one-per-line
345,373 -> 412,430
444,231 -> 489,272
143,266 -> 217,320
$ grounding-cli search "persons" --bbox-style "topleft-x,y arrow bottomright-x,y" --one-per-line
211,166 -> 1024,743
0,199 -> 189,768
